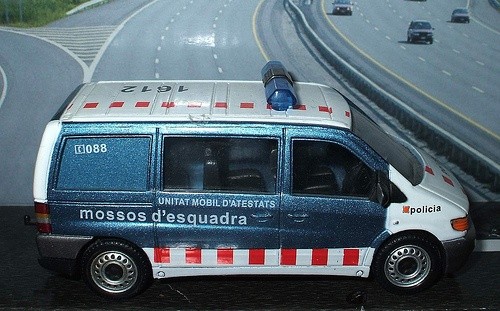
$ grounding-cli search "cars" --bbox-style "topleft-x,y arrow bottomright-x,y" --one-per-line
450,8 -> 470,23
331,0 -> 353,15
407,19 -> 435,44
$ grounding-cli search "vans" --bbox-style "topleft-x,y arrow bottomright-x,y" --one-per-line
23,60 -> 476,301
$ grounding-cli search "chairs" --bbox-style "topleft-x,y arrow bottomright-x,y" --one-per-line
162,161 -> 191,190
272,148 -> 333,195
203,144 -> 266,193
